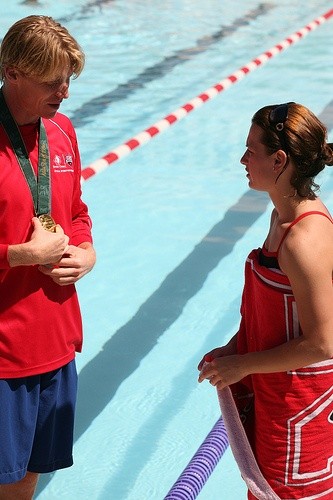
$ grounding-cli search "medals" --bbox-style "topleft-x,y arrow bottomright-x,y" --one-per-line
36,213 -> 59,236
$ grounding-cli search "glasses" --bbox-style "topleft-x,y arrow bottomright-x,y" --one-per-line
269,101 -> 296,151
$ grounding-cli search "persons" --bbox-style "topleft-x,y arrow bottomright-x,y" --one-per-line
184,101 -> 333,498
1,13 -> 104,499
